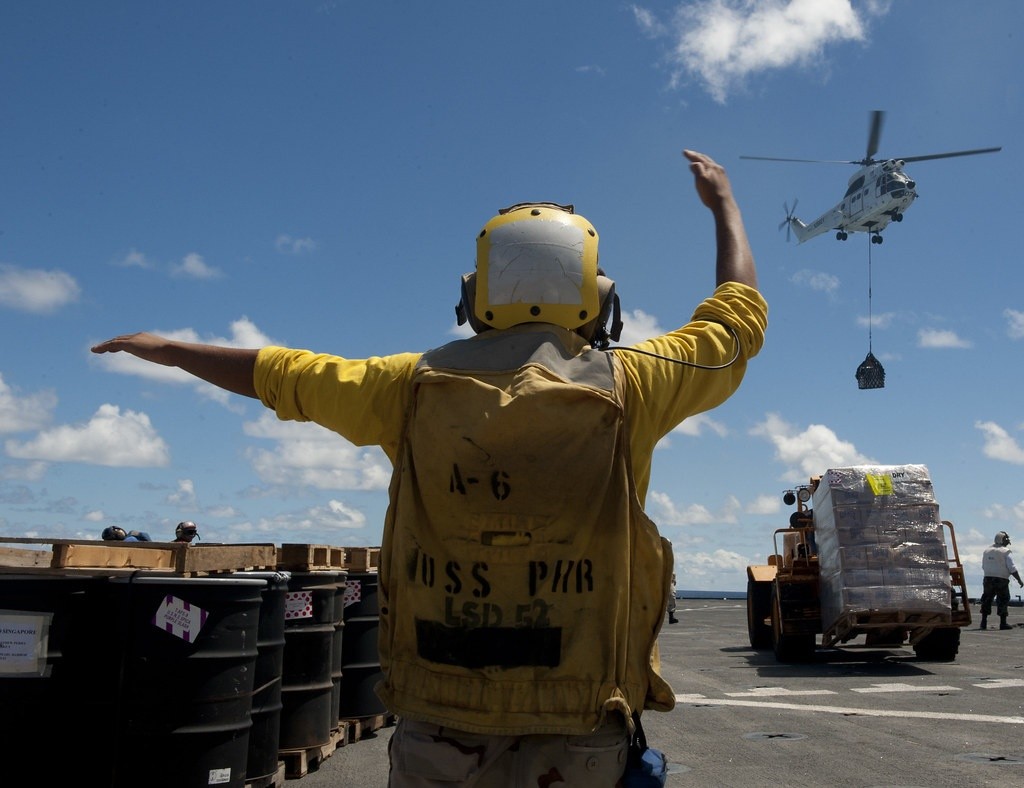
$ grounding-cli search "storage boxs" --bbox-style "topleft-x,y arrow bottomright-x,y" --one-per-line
813,465 -> 954,629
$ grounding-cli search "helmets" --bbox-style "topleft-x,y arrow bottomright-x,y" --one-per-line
994,531 -> 1011,546
455,201 -> 615,344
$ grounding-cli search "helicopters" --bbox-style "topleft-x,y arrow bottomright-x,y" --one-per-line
734,110 -> 1003,248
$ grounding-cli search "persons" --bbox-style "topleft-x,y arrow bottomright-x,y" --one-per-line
90,150 -> 767,788
102,521 -> 198,543
667,573 -> 679,624
981,532 -> 1024,630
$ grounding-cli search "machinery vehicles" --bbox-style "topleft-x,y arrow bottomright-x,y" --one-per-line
745,474 -> 972,665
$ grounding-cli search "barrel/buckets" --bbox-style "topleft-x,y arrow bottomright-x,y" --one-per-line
0,564 -> 388,788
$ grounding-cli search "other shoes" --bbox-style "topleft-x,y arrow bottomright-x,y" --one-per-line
999,623 -> 1012,629
980,621 -> 987,629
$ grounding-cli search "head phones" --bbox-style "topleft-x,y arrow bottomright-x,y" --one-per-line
461,268 -> 615,347
